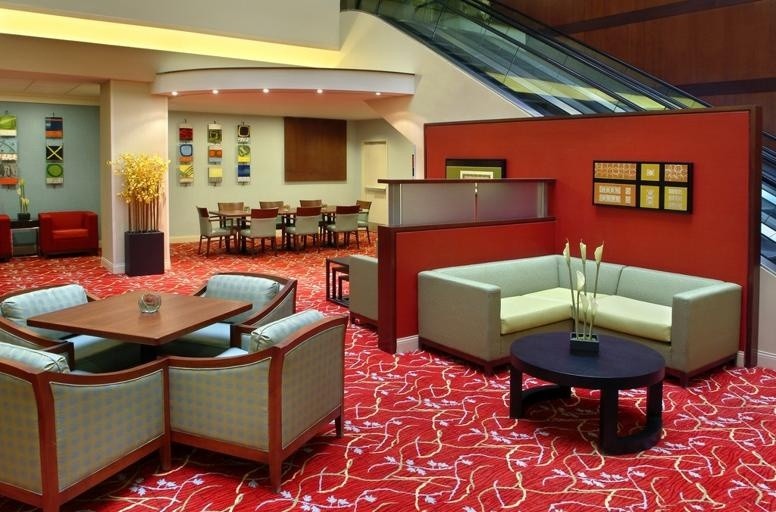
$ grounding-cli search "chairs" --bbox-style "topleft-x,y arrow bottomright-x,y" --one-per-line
0,215 -> 11,258
38,211 -> 99,259
196,199 -> 372,257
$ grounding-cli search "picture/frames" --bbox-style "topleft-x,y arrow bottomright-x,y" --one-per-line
445,159 -> 507,179
592,160 -> 693,213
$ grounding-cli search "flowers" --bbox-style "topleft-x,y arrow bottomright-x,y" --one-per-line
16,179 -> 29,213
563,238 -> 605,339
104,152 -> 171,234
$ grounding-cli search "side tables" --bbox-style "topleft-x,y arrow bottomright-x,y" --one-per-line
12,220 -> 38,257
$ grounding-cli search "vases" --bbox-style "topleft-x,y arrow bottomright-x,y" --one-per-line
124,230 -> 164,276
569,333 -> 600,356
17,213 -> 30,220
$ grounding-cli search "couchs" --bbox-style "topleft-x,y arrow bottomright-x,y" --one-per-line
417,255 -> 742,386
348,254 -> 378,326
